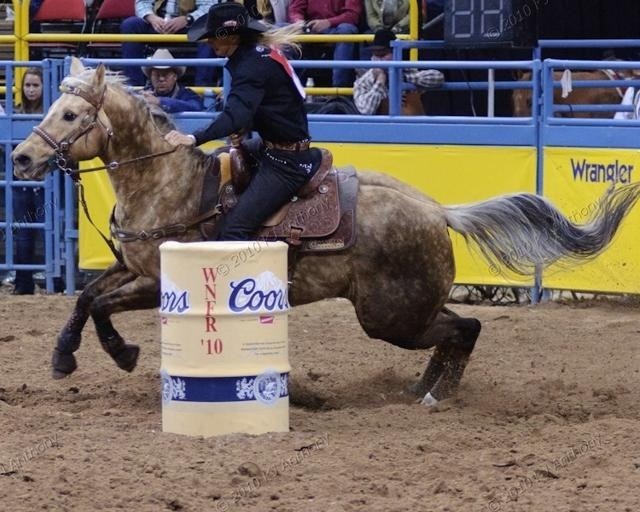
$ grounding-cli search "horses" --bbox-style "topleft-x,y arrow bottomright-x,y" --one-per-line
10,56 -> 640,407
512,69 -> 640,119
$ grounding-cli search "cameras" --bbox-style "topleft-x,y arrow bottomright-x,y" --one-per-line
303,25 -> 312,33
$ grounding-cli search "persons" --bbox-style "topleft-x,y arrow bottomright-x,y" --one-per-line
120,0 -> 444,115
162,2 -> 322,241
2,67 -> 48,289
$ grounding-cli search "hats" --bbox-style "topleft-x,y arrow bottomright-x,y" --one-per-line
141,48 -> 187,78
188,2 -> 268,42
364,30 -> 396,51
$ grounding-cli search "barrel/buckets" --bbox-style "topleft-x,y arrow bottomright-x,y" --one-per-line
158,241 -> 292,436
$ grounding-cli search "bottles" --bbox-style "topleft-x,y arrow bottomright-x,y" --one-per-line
306,78 -> 315,103
203,88 -> 216,112
163,13 -> 171,25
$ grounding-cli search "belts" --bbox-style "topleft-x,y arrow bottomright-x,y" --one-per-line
262,138 -> 311,152
